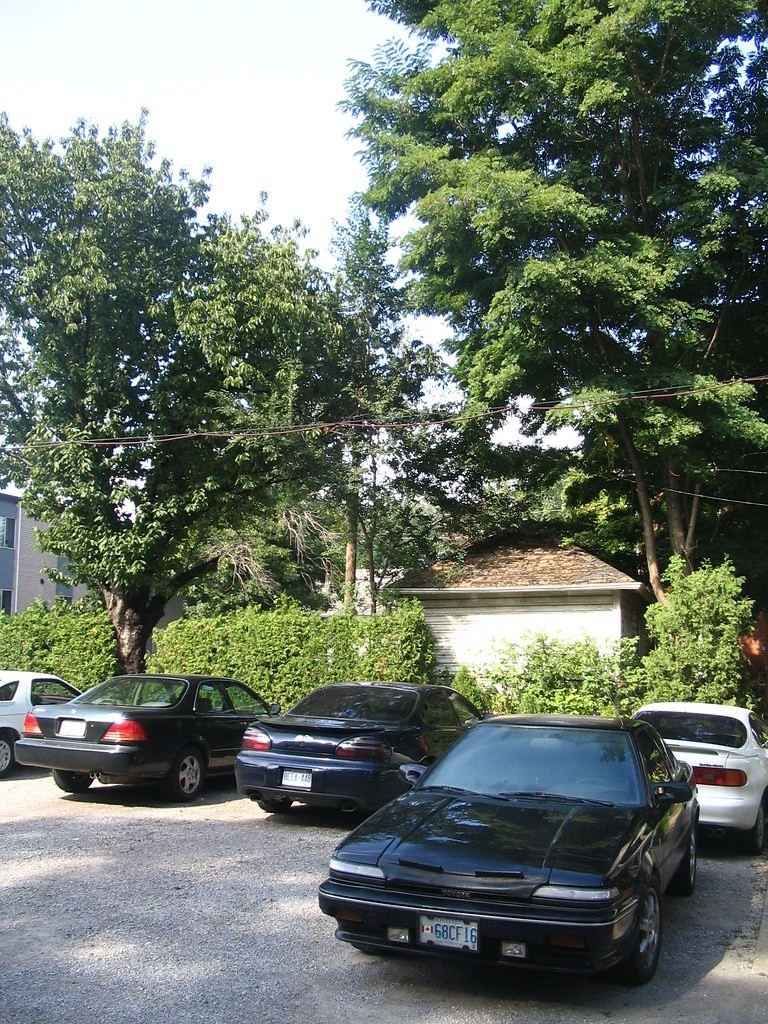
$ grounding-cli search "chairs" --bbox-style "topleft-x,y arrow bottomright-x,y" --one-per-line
195,698 -> 213,710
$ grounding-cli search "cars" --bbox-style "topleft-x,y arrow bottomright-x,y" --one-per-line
0,668 -> 85,779
629,702 -> 768,856
235,681 -> 493,813
13,673 -> 282,804
317,714 -> 701,986
